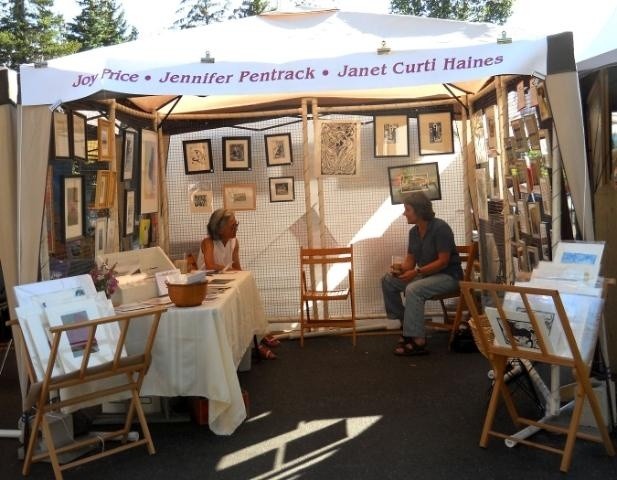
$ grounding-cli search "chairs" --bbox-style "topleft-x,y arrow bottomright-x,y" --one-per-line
297,245 -> 357,347
423,241 -> 478,350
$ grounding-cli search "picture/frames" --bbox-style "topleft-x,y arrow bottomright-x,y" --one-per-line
63,173 -> 87,244
123,128 -> 160,236
51,105 -> 90,161
268,177 -> 295,203
223,183 -> 257,212
372,111 -> 455,158
388,161 -> 443,206
182,131 -> 294,175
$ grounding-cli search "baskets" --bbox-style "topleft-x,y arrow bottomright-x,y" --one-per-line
469,313 -> 495,359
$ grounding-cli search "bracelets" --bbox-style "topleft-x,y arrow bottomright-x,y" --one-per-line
414,261 -> 425,278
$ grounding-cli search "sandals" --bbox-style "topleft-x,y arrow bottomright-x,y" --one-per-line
393,337 -> 427,355
263,335 -> 280,347
257,345 -> 277,359
399,333 -> 413,344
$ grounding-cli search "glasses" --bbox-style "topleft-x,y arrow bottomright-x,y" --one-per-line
230,222 -> 239,228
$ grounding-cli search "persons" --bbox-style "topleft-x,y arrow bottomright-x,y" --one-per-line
193,208 -> 281,364
431,123 -> 440,141
381,191 -> 464,354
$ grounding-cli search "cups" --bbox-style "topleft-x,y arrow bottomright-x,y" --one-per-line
390,255 -> 404,276
175,259 -> 188,274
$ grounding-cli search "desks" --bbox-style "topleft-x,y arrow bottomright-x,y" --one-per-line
111,270 -> 256,424
